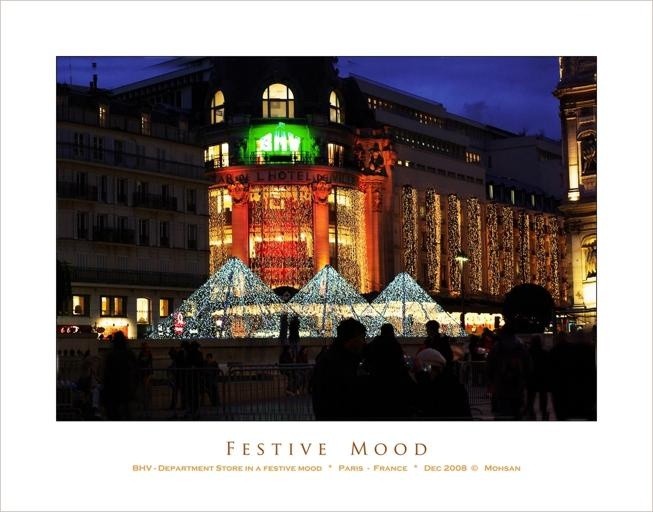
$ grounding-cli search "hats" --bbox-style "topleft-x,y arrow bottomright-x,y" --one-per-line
410,347 -> 446,375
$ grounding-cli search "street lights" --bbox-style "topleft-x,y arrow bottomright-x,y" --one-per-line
454,255 -> 469,332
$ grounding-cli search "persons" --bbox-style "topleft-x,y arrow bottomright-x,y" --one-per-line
56,314 -> 596,419
